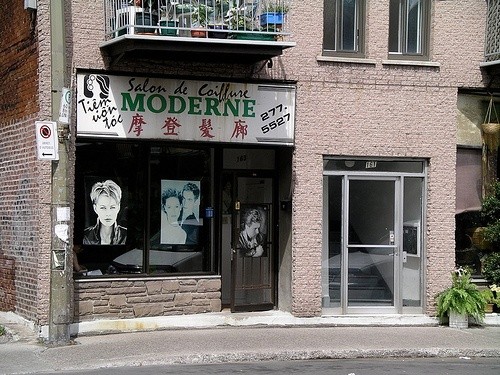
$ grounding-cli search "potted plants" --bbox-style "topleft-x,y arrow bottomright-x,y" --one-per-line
258,4 -> 289,27
207,0 -> 228,38
434,266 -> 487,329
479,290 -> 500,312
188,4 -> 212,36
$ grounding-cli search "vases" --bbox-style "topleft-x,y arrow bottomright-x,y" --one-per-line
136,12 -> 160,33
159,21 -> 179,35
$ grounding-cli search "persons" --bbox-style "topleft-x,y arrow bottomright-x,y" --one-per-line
161,183 -> 199,244
239,208 -> 266,257
84,179 -> 127,245
